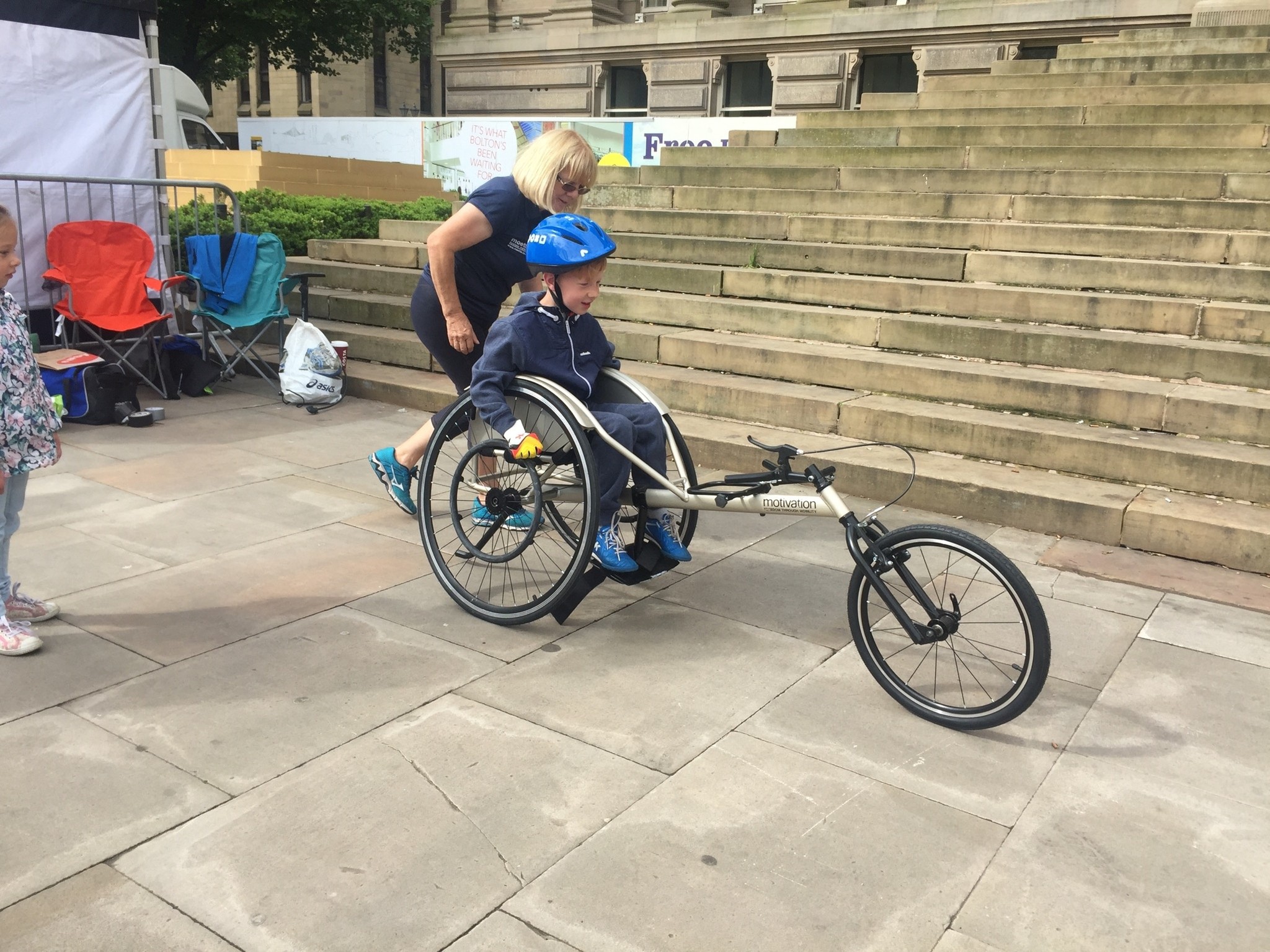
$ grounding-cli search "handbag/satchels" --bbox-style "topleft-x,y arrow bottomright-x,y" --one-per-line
153,334 -> 223,399
38,363 -> 141,427
280,317 -> 342,405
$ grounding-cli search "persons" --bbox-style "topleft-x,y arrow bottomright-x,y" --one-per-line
471,213 -> 693,574
0,205 -> 58,655
367,130 -> 596,532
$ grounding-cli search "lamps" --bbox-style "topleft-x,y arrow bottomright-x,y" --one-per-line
896,0 -> 910,5
513,16 -> 522,29
635,12 -> 645,23
399,102 -> 409,117
410,103 -> 420,117
753,3 -> 766,14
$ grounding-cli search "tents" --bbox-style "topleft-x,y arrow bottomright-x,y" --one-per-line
0,0 -> 182,337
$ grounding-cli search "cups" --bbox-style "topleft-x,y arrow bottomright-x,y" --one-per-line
331,341 -> 349,369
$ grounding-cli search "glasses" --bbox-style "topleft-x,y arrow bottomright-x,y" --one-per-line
556,172 -> 590,196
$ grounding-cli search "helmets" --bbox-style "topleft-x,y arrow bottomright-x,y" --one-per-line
527,214 -> 615,268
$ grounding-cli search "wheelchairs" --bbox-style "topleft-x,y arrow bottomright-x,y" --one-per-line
414,362 -> 1051,733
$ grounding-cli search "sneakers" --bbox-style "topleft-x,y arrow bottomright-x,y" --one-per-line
368,447 -> 418,516
589,512 -> 640,571
473,496 -> 545,532
644,512 -> 692,561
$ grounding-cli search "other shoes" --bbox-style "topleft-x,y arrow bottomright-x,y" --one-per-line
5,582 -> 60,622
1,622 -> 44,656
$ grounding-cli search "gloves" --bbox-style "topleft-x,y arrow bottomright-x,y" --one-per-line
503,420 -> 543,459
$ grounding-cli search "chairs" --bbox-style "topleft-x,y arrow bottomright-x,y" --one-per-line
176,232 -> 301,395
40,220 -> 187,401
